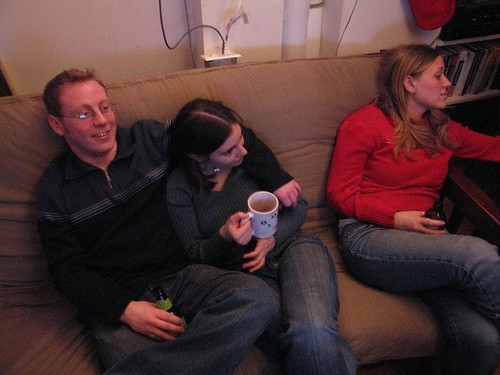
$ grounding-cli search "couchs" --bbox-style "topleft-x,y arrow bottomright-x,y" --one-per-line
0,51 -> 500,375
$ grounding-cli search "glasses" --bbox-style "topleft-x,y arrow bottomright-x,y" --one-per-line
60,103 -> 117,120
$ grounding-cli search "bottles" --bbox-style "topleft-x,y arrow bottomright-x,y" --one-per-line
425,200 -> 446,230
153,287 -> 188,330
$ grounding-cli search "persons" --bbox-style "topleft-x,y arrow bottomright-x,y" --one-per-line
33,65 -> 302,375
328,43 -> 500,375
164,98 -> 358,375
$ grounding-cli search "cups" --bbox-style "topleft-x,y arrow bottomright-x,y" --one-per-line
240,191 -> 279,238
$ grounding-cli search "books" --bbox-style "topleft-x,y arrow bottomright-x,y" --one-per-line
435,41 -> 500,99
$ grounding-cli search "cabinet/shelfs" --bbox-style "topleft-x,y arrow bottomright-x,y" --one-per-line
436,34 -> 500,106
